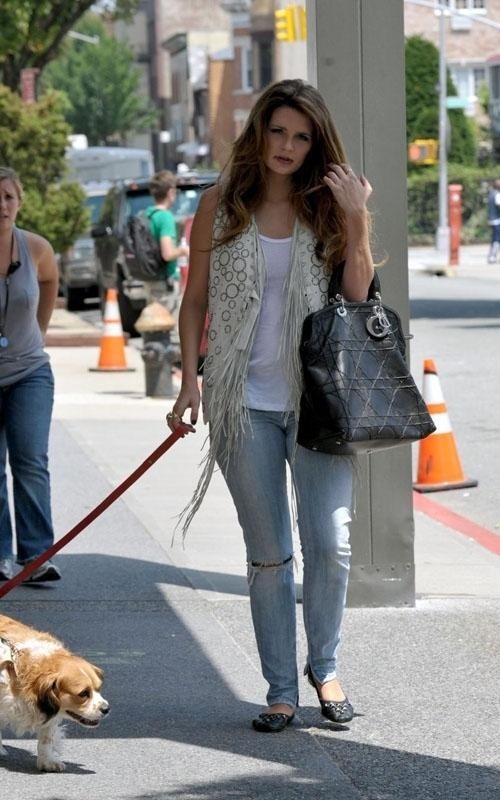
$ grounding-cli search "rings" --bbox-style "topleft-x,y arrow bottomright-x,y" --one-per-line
166,413 -> 174,420
171,411 -> 180,419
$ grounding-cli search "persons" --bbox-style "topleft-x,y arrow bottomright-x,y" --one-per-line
167,77 -> 378,734
0,167 -> 62,584
486,177 -> 500,264
140,168 -> 189,315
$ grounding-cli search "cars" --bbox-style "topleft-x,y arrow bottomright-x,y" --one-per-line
51,129 -> 236,336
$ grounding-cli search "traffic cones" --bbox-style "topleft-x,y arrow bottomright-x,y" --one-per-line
87,290 -> 137,374
412,356 -> 481,497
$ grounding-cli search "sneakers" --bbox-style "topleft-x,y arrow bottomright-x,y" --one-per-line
18,556 -> 62,584
0,557 -> 15,582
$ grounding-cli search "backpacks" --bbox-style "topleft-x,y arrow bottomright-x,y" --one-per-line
120,212 -> 167,280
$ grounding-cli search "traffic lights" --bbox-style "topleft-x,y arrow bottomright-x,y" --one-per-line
408,140 -> 430,164
275,6 -> 295,43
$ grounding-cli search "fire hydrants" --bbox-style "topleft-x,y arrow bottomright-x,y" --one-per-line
130,305 -> 179,400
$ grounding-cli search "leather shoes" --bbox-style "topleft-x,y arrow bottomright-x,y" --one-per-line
307,664 -> 354,725
251,697 -> 300,733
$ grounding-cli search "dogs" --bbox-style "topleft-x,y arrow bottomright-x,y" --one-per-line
0,614 -> 113,775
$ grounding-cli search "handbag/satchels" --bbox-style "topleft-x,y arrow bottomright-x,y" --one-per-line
293,257 -> 438,461
